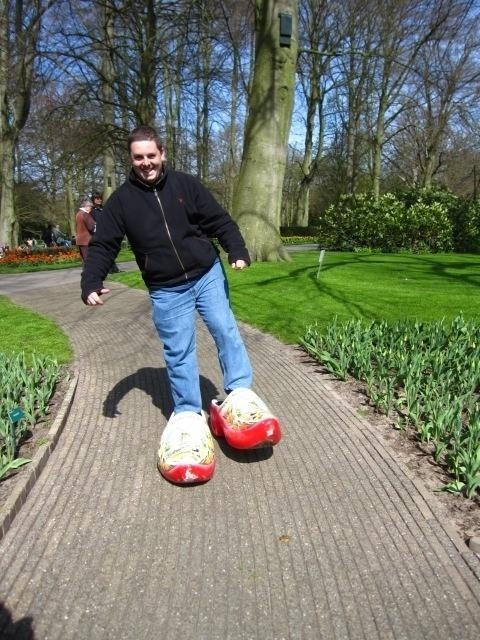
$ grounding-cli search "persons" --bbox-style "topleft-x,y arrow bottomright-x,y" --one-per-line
18,223 -> 76,250
89,192 -> 121,273
78,126 -> 282,485
74,200 -> 97,260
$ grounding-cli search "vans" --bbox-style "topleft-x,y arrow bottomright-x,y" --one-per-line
210,387 -> 280,450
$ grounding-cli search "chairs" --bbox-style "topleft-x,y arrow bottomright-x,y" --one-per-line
157,409 -> 215,485
